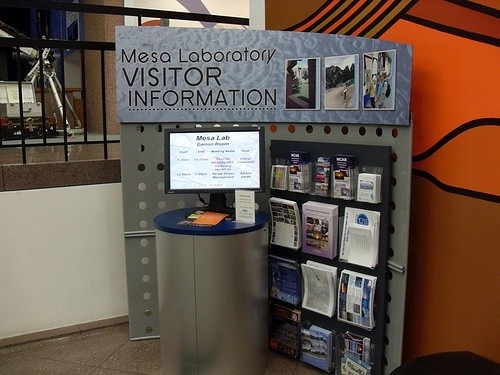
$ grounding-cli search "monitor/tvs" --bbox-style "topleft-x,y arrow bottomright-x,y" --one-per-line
163,126 -> 265,194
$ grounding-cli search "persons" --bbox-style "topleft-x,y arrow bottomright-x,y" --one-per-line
367,72 -> 390,108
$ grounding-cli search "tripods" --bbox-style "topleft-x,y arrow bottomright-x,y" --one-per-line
29,58 -> 83,138
